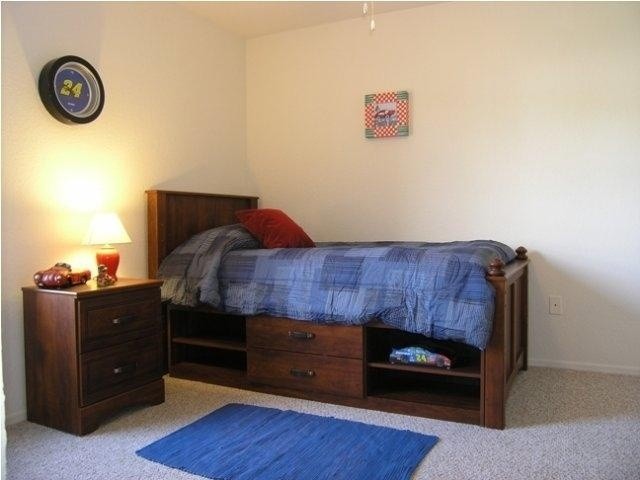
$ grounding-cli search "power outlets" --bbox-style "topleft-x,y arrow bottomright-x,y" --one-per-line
549,295 -> 563,315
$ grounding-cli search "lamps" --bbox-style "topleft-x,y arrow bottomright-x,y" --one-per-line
80,212 -> 132,282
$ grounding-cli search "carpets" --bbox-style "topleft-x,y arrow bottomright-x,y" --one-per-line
135,403 -> 440,480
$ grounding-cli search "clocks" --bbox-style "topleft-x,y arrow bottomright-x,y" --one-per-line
38,55 -> 105,126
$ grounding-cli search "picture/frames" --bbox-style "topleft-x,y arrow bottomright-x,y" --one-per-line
364,91 -> 409,138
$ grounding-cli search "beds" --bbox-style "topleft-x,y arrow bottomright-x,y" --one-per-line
146,190 -> 529,429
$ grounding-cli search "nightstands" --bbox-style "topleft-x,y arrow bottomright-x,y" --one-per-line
21,275 -> 165,436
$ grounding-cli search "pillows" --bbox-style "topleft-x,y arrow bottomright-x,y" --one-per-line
233,208 -> 315,248
